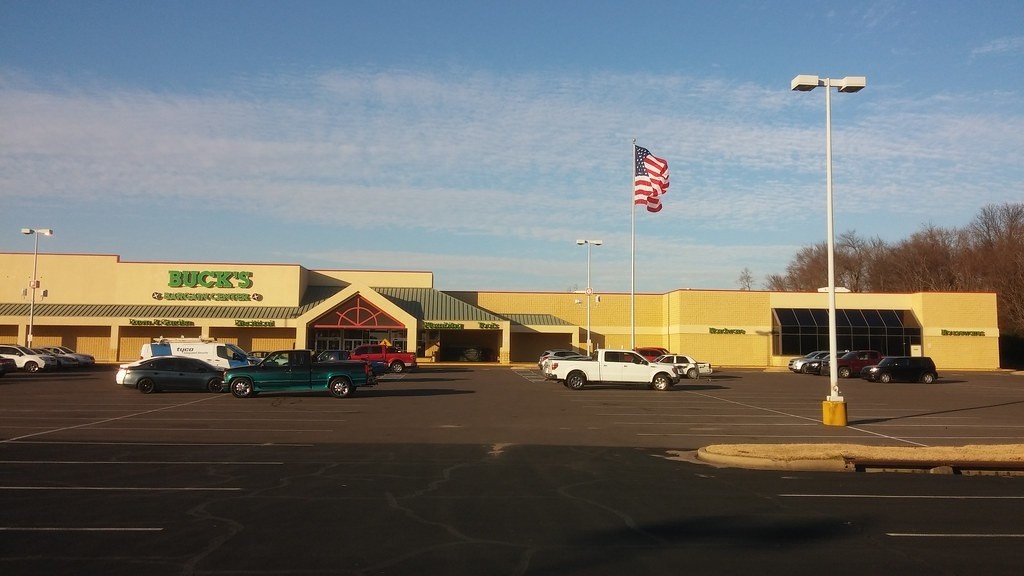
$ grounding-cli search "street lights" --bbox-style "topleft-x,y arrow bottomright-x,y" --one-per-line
21,227 -> 55,348
577,239 -> 603,356
791,73 -> 866,428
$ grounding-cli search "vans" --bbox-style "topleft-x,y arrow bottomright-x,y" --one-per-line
138,336 -> 263,373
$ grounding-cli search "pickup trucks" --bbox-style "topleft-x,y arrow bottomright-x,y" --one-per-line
635,347 -> 669,363
317,350 -> 390,376
349,343 -> 418,374
221,349 -> 378,397
547,348 -> 680,392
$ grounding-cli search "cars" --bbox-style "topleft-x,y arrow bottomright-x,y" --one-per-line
787,350 -> 939,384
650,353 -> 713,380
247,350 -> 271,360
0,341 -> 95,374
114,357 -> 223,395
461,344 -> 483,361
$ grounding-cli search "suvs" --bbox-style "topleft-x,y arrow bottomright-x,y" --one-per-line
538,349 -> 591,377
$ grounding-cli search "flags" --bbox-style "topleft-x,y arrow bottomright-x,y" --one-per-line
635,146 -> 670,212
